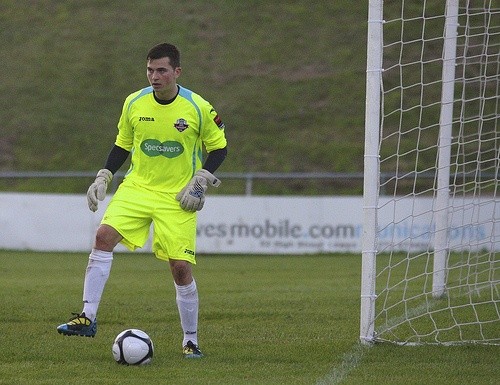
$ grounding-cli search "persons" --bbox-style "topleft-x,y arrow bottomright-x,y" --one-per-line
56,43 -> 227,359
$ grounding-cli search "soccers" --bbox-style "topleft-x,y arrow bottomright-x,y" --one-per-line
113,329 -> 152,366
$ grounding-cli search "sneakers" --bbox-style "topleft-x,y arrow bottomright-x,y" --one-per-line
182,339 -> 204,359
57,312 -> 97,337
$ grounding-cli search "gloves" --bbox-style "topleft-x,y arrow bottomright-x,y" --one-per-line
176,169 -> 222,213
86,169 -> 114,212
